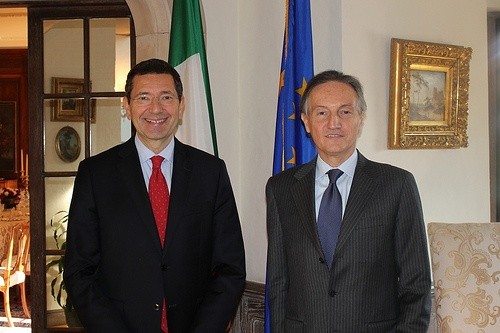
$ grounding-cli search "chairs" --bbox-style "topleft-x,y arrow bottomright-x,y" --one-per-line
427,221 -> 500,333
0,220 -> 30,329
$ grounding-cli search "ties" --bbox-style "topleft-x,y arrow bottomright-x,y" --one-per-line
147,155 -> 168,333
316,169 -> 345,272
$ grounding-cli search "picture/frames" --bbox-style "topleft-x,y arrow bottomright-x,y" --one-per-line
50,77 -> 93,122
388,38 -> 474,149
55,126 -> 81,163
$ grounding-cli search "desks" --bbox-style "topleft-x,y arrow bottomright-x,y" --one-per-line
0,202 -> 30,267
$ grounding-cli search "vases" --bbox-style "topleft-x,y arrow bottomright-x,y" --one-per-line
4,204 -> 13,209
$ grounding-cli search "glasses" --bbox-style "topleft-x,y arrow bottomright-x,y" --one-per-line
128,95 -> 179,107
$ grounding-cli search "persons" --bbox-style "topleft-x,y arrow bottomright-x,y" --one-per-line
265,70 -> 431,333
63,58 -> 248,332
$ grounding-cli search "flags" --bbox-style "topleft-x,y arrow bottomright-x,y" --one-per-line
265,0 -> 315,333
165,0 -> 219,156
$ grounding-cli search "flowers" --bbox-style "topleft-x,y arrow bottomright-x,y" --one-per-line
0,188 -> 21,209
